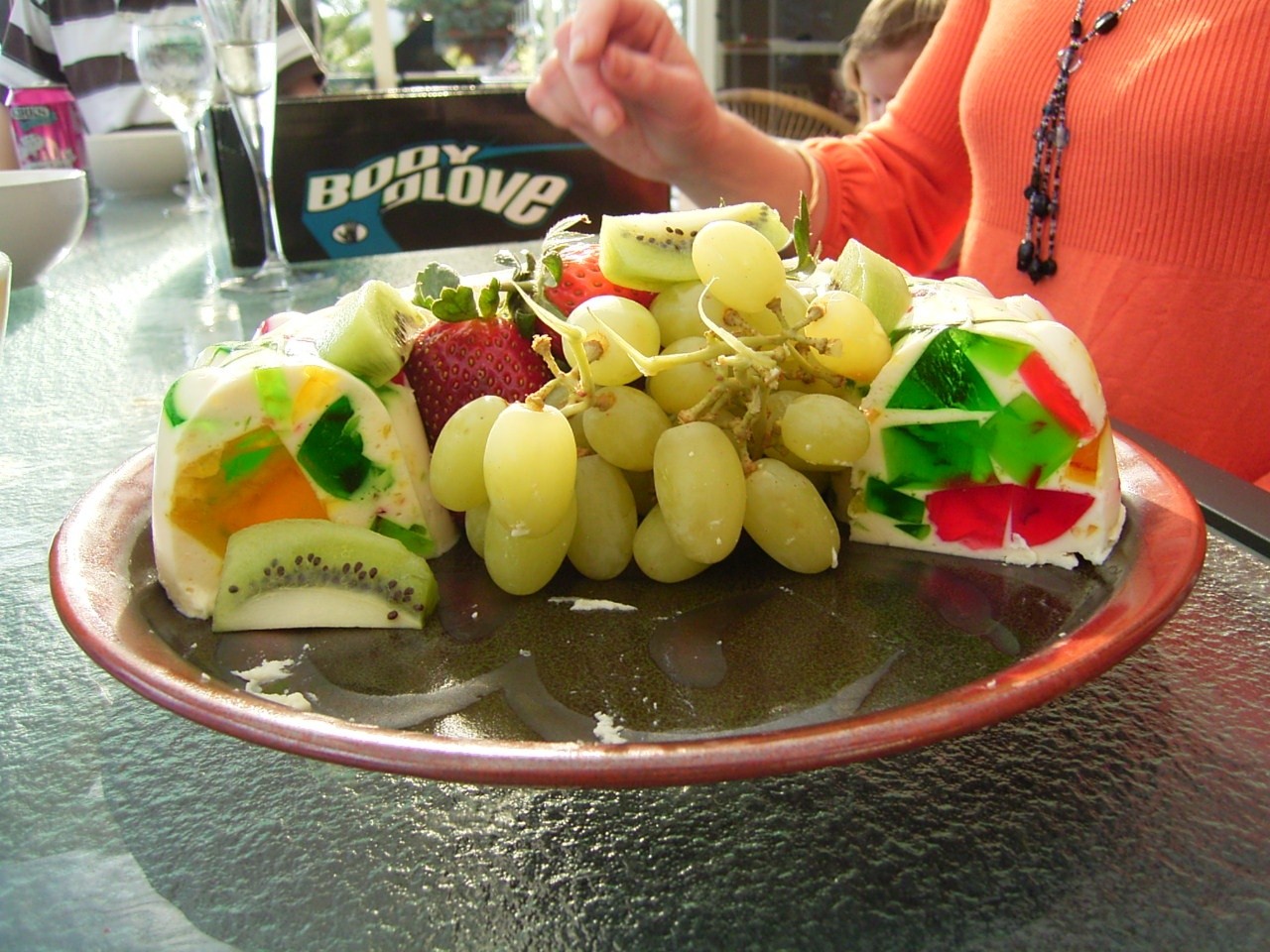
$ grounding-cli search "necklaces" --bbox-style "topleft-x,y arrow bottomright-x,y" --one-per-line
1015,0 -> 1136,284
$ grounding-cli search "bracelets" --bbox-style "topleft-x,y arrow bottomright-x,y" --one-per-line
793,141 -> 819,215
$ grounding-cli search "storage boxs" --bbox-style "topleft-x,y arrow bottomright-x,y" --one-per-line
212,80 -> 665,266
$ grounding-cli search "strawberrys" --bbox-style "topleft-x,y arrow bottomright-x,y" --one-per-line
495,214 -> 658,361
405,262 -> 554,455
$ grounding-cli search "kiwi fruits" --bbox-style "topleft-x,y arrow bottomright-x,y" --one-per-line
316,280 -> 429,387
597,202 -> 793,293
828,237 -> 911,337
212,518 -> 440,633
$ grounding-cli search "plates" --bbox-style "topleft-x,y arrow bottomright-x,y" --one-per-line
47,428 -> 1207,788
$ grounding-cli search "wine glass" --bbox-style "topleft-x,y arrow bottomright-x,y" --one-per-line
131,19 -> 220,216
196,1 -> 336,293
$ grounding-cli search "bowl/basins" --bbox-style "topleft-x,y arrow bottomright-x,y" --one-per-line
86,130 -> 195,198
0,169 -> 89,288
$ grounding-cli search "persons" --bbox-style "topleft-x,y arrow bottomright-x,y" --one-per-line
837,0 -> 965,283
0,0 -> 325,138
525,0 -> 1270,491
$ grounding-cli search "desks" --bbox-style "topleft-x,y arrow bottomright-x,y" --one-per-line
2,193 -> 1270,951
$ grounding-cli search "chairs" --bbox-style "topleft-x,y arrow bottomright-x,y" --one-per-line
713,86 -> 855,140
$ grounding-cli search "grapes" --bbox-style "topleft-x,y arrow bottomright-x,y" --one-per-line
431,220 -> 892,595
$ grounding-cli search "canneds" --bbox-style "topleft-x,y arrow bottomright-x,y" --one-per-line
6,78 -> 104,216
